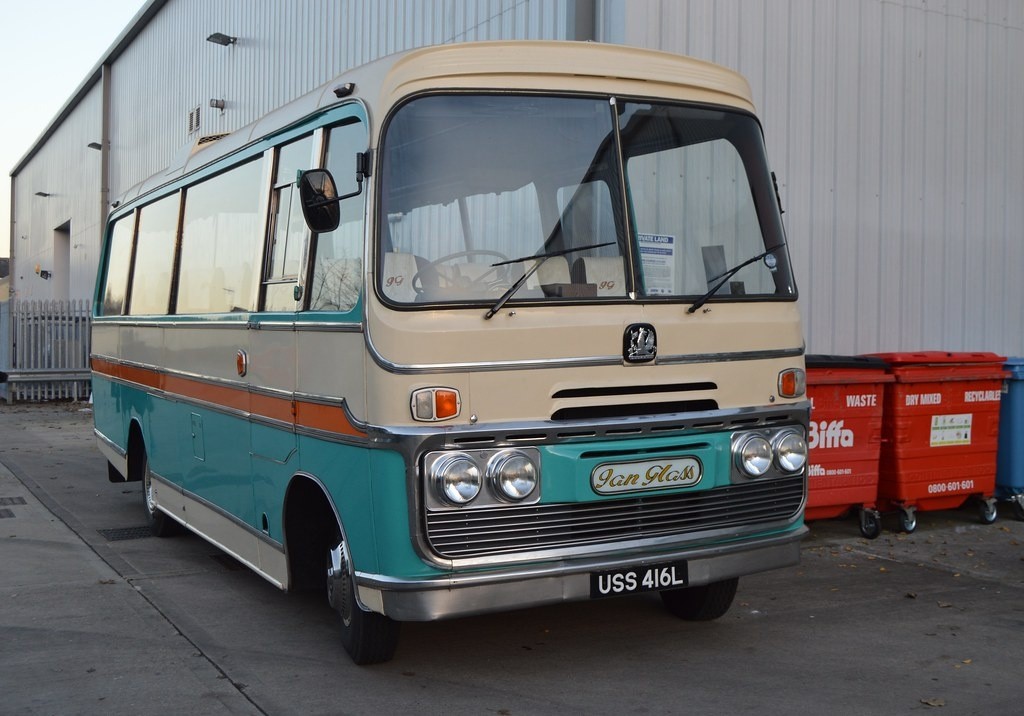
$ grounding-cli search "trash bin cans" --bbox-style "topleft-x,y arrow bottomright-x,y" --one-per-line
806,351 -> 1024,539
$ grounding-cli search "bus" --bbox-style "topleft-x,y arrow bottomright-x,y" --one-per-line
92,40 -> 814,668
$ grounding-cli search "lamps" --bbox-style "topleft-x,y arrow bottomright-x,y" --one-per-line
35,192 -> 50,197
40,270 -> 51,280
207,32 -> 236,46
88,142 -> 101,150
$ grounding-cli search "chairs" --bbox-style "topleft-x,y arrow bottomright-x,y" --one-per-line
511,255 -> 571,290
574,258 -> 627,297
452,263 -> 506,292
135,254 -> 437,312
